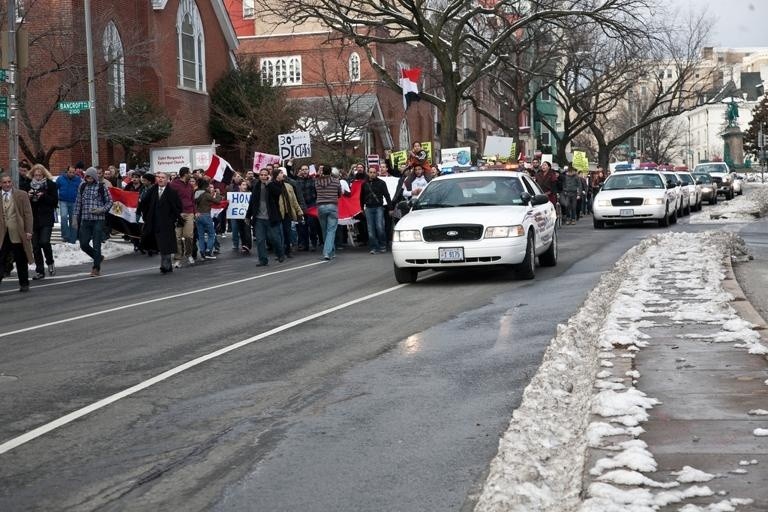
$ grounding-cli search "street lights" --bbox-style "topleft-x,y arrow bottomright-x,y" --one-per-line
530,49 -> 590,169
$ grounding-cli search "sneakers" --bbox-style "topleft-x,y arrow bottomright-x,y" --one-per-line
20,285 -> 29,292
90,229 -> 388,278
47,260 -> 56,276
33,271 -> 46,280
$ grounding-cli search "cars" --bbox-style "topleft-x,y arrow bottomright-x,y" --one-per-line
590,159 -> 740,229
392,163 -> 560,283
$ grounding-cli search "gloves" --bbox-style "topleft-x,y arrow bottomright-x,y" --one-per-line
90,207 -> 107,215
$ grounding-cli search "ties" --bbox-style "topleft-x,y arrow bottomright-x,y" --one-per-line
4,193 -> 9,208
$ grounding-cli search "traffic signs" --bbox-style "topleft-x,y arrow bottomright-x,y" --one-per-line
0,67 -> 10,120
58,99 -> 92,116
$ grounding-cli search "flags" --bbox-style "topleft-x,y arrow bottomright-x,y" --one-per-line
400,68 -> 422,112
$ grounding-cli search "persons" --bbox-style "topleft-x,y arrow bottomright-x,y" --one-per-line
514,159 -> 607,225
2,141 -> 441,291
